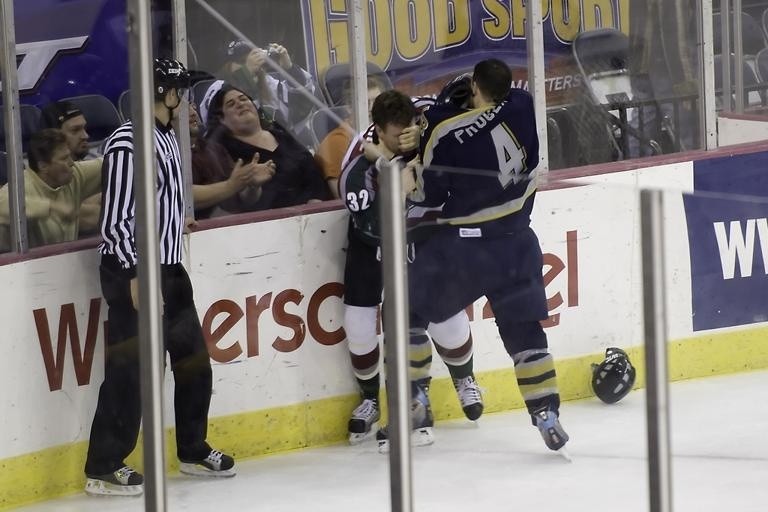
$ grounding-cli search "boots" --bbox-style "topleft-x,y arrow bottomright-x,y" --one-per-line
374,376 -> 435,441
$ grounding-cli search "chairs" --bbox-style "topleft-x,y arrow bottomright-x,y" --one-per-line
3,10 -> 766,189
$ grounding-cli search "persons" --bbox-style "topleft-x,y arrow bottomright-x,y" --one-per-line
2,41 -> 390,255
79,59 -> 236,497
338,57 -> 571,452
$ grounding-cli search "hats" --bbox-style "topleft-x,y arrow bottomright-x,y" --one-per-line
39,100 -> 83,129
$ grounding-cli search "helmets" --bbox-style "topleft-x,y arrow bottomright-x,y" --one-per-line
588,346 -> 637,406
153,55 -> 192,101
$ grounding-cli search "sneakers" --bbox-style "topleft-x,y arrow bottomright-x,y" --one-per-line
175,448 -> 235,473
348,398 -> 382,434
528,409 -> 568,451
84,465 -> 143,488
449,374 -> 485,422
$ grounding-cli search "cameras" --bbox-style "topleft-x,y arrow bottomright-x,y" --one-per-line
261,50 -> 275,57
